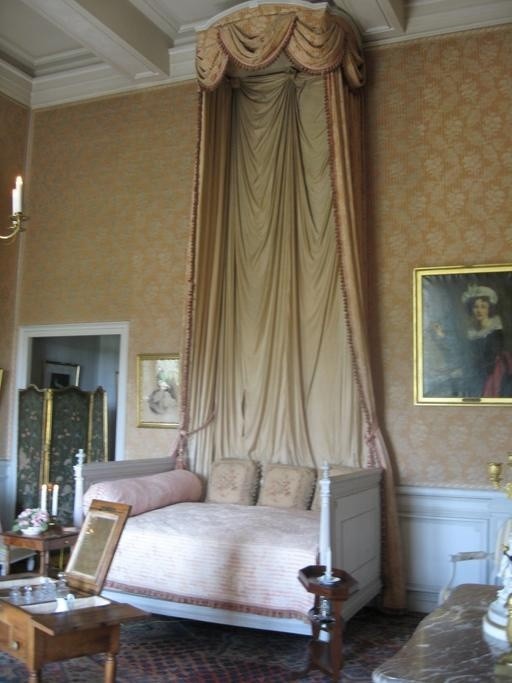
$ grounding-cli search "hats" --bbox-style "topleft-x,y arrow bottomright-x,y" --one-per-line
461,286 -> 498,304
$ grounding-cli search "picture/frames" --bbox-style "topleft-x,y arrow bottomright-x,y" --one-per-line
135,350 -> 185,429
41,360 -> 82,391
411,263 -> 512,406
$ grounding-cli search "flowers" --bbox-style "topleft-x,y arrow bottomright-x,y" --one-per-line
11,507 -> 50,534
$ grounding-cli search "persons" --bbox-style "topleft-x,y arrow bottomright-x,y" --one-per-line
430,285 -> 510,397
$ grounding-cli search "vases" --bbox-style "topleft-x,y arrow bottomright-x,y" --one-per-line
21,524 -> 48,537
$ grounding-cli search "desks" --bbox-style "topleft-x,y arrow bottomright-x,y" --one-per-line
1,526 -> 80,577
2,497 -> 150,682
369,581 -> 512,682
292,562 -> 359,681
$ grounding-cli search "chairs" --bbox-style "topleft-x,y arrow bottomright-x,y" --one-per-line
436,517 -> 512,607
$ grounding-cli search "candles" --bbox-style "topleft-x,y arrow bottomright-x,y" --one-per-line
15,174 -> 26,213
12,189 -> 19,217
50,484 -> 60,516
326,545 -> 333,575
38,484 -> 48,511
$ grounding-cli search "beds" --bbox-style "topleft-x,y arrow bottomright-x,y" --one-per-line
71,421 -> 389,642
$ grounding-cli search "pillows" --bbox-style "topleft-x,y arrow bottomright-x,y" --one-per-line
254,461 -> 315,511
203,454 -> 260,505
308,464 -> 361,511
